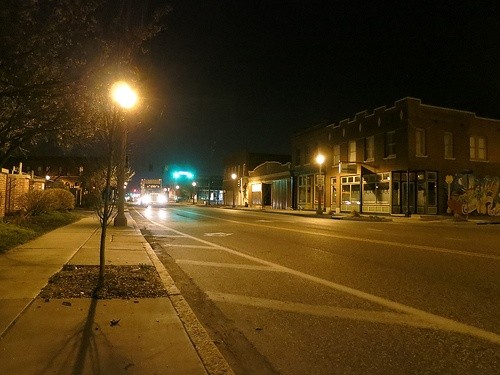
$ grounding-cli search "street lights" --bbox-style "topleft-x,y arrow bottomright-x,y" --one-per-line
113,84 -> 134,226
44,175 -> 50,190
191,181 -> 197,204
316,154 -> 324,215
230,173 -> 238,208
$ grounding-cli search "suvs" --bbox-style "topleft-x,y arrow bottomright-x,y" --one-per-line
142,187 -> 167,209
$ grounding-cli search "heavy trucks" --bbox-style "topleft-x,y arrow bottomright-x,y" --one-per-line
139,178 -> 162,196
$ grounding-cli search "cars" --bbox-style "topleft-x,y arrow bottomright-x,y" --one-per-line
123,188 -> 224,207
